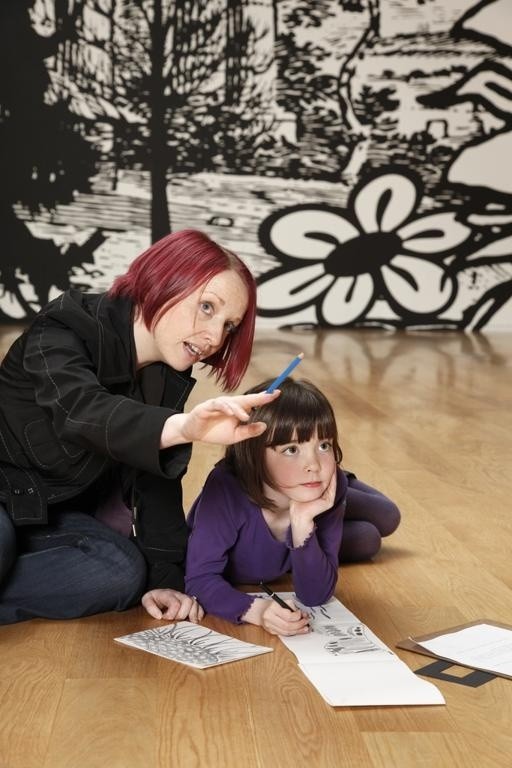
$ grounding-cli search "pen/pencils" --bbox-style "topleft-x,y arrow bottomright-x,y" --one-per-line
252,352 -> 305,410
256,581 -> 314,633
